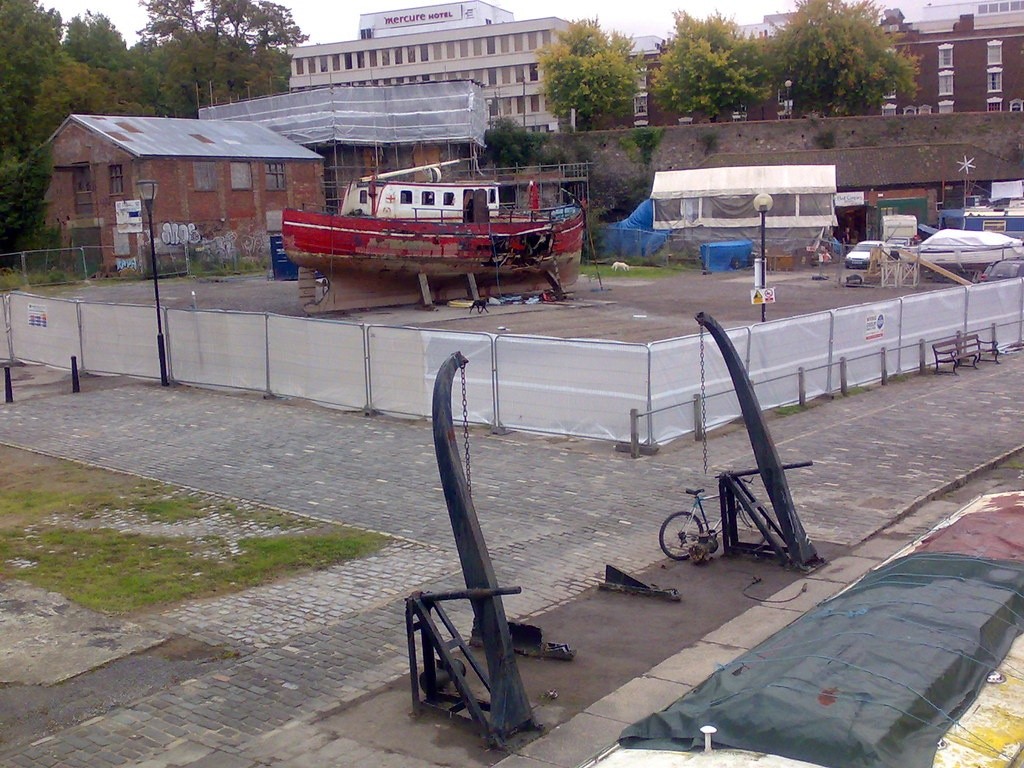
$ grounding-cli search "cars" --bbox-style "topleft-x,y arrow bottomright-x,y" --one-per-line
845,239 -> 892,269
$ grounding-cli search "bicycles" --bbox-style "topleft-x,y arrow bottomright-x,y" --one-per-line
657,482 -> 772,561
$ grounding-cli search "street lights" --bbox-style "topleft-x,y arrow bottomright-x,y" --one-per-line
754,192 -> 772,323
785,80 -> 792,119
136,178 -> 172,387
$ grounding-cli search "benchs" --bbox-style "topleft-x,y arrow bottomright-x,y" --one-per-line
932,334 -> 1001,375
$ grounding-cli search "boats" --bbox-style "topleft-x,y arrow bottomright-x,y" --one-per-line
281,167 -> 592,314
895,241 -> 1023,281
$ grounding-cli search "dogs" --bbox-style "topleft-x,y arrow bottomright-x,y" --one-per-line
469,298 -> 489,314
610,262 -> 630,272
